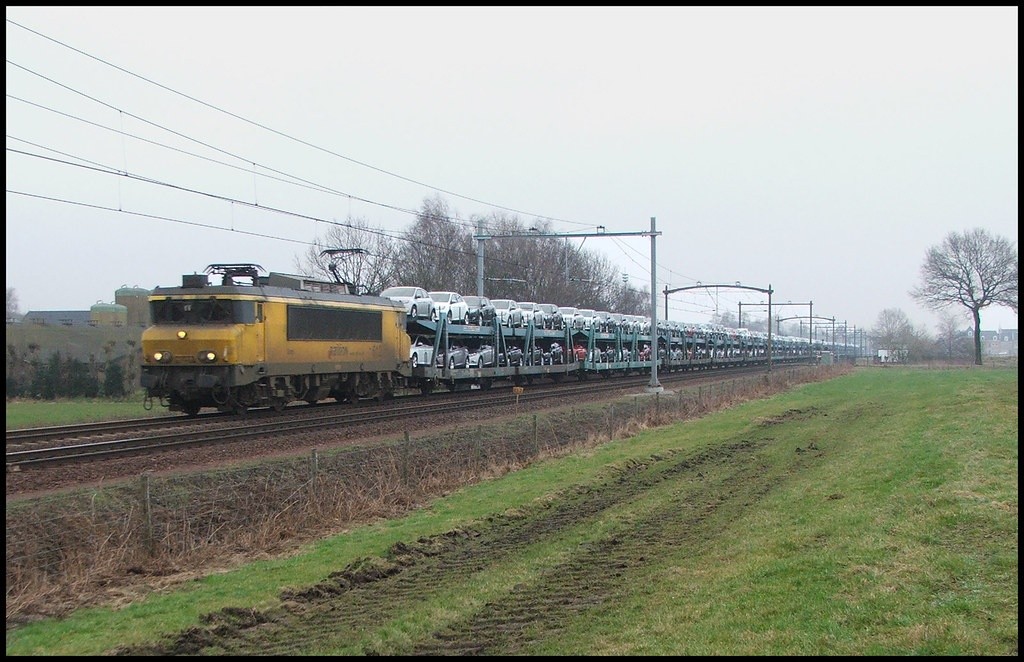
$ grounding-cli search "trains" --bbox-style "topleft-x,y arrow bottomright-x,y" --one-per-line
136,242 -> 866,417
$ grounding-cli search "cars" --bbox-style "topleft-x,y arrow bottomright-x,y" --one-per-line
499,344 -> 524,367
436,337 -> 470,369
469,344 -> 495,369
427,291 -> 471,326
409,335 -> 438,369
462,295 -> 496,326
517,300 -> 866,366
379,286 -> 437,322
489,299 -> 525,329
